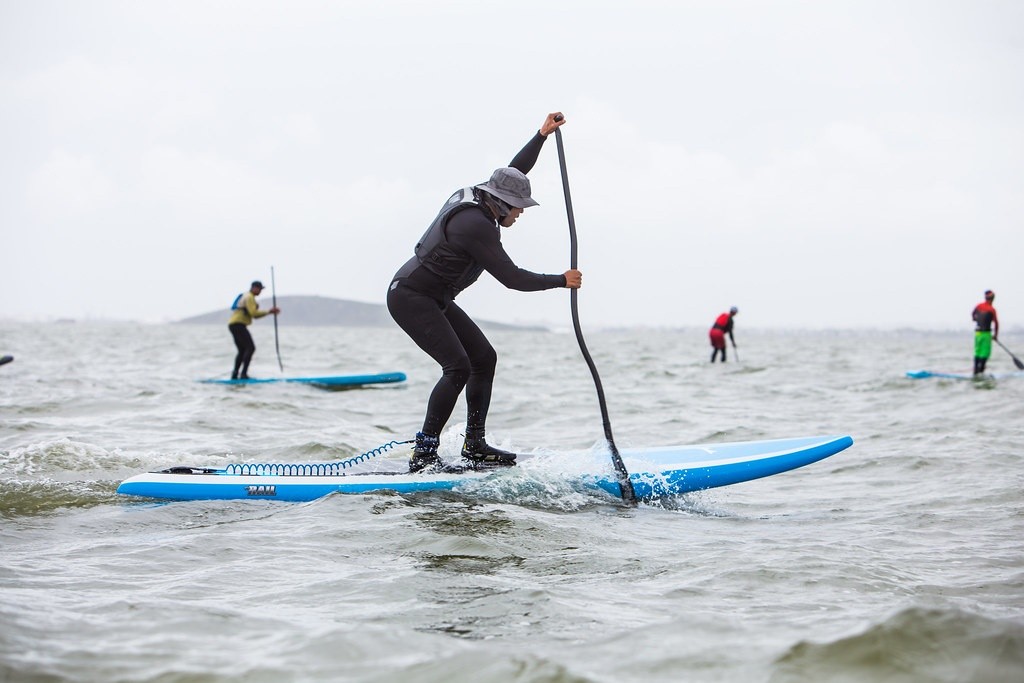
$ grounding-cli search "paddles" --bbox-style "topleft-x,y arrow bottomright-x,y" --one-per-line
990,335 -> 1024,370
271,265 -> 284,371
551,111 -> 638,505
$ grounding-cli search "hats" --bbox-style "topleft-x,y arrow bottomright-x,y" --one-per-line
476,167 -> 539,208
985,291 -> 994,299
252,281 -> 265,289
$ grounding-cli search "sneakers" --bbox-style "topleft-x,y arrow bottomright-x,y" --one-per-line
409,446 -> 463,473
460,434 -> 517,465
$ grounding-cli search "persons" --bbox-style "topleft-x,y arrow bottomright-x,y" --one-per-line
387,113 -> 583,474
710,306 -> 738,361
228,281 -> 281,379
972,290 -> 999,374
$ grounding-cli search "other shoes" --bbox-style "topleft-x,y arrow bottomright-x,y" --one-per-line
232,371 -> 238,378
241,372 -> 248,378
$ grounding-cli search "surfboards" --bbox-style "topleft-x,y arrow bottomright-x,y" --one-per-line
195,371 -> 408,387
906,369 -> 973,380
115,438 -> 855,502
